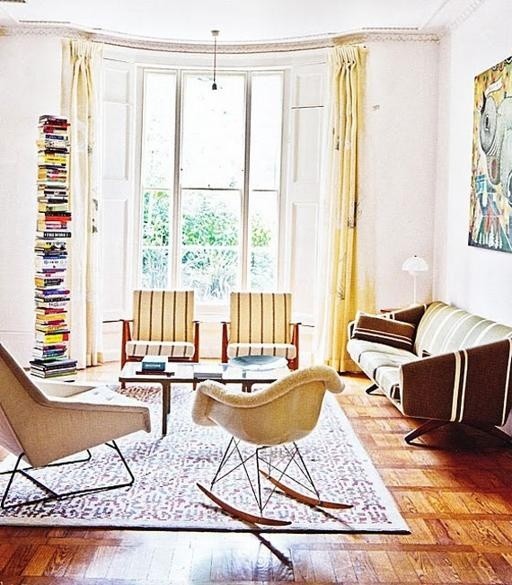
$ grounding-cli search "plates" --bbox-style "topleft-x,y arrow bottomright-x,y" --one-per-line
229,353 -> 289,371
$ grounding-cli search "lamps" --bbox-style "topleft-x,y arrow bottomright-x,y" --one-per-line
207,28 -> 221,93
401,254 -> 432,307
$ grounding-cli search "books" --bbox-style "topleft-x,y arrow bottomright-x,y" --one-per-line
136,353 -> 222,379
28,114 -> 79,378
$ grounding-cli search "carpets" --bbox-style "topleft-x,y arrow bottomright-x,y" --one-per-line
0,365 -> 415,536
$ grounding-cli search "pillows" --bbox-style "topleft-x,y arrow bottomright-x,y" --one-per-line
354,311 -> 417,355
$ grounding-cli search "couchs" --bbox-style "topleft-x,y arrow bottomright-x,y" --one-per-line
346,301 -> 512,453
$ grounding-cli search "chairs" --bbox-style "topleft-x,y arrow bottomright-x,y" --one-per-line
220,291 -> 306,392
190,366 -> 361,528
104,291 -> 202,388
0,340 -> 153,510
